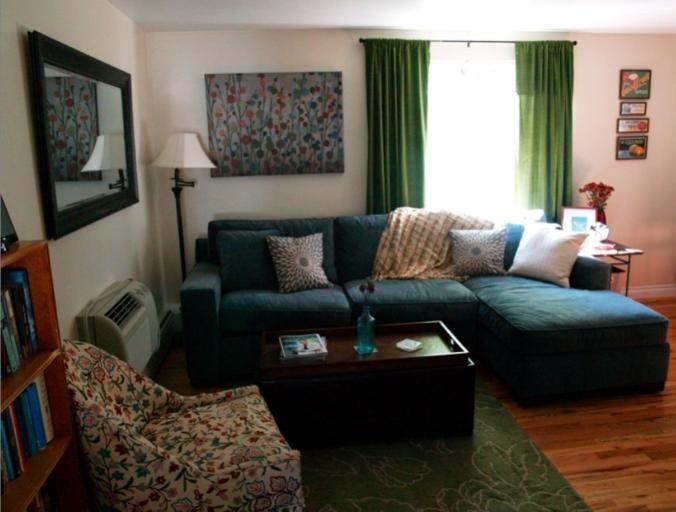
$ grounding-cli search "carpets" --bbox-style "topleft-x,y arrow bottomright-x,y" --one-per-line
291,366 -> 592,512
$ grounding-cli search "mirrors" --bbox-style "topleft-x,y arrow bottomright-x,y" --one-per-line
28,28 -> 139,241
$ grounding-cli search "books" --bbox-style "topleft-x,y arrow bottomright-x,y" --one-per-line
0,265 -> 54,511
278,333 -> 328,365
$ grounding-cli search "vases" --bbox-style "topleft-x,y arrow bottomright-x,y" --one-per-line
597,209 -> 606,225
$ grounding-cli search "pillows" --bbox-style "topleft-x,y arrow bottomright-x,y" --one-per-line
219,228 -> 338,294
448,222 -> 588,289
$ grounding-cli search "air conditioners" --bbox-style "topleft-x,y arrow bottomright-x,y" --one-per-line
75,276 -> 163,375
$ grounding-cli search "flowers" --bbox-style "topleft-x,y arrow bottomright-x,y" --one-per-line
360,280 -> 374,326
579,182 -> 614,209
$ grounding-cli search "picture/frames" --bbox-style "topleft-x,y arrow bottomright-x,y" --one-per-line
560,206 -> 599,231
616,69 -> 652,161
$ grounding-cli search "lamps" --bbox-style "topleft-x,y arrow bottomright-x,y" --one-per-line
80,134 -> 130,191
151,131 -> 217,284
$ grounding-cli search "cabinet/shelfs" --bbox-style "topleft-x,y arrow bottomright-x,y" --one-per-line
0,239 -> 84,511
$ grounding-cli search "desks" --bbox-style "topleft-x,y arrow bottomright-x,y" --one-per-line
590,240 -> 644,296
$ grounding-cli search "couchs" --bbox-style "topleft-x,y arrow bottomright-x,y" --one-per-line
179,214 -> 670,409
62,335 -> 305,511
